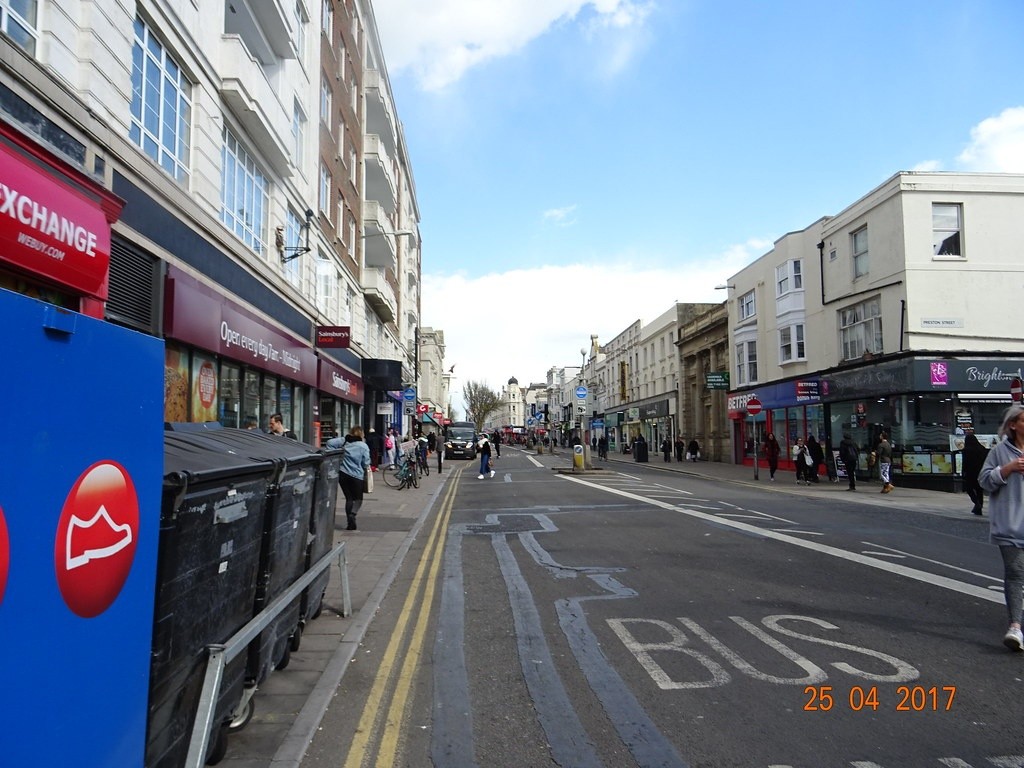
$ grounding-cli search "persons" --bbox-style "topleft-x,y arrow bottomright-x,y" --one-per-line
978,404 -> 1024,652
518,434 -> 580,450
675,438 -> 684,462
632,433 -> 645,449
955,407 -> 973,416
244,413 -> 297,440
326,425 -> 370,530
876,431 -> 895,494
792,437 -> 811,486
763,433 -> 780,482
688,437 -> 699,462
903,455 -> 917,472
367,427 -> 445,469
478,434 -> 495,479
663,436 -> 672,463
805,435 -> 824,483
963,434 -> 988,515
839,432 -> 860,491
493,430 -> 500,459
592,435 -> 606,453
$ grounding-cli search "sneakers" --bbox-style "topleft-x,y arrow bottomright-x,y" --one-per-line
1003,627 -> 1024,652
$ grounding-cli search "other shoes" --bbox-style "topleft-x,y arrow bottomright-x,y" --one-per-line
881,483 -> 894,493
771,475 -> 777,481
972,507 -> 982,516
490,470 -> 495,478
346,513 -> 357,530
390,465 -> 396,469
477,474 -> 484,479
796,480 -> 811,486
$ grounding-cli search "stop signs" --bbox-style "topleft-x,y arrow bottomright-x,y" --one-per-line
746,399 -> 762,414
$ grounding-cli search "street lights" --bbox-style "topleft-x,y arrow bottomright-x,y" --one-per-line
580,347 -> 588,386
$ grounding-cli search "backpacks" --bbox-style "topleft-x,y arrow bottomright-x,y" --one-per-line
385,437 -> 392,450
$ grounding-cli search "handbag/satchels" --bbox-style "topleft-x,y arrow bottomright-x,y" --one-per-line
697,451 -> 700,458
685,452 -> 691,460
804,453 -> 813,467
363,466 -> 374,493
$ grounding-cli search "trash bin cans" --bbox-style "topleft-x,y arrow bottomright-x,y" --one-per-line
634,441 -> 648,463
145,426 -> 345,763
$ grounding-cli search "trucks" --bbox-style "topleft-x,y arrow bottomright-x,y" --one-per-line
443,421 -> 478,460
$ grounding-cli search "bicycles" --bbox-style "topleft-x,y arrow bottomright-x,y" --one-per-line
383,451 -> 422,490
415,450 -> 429,479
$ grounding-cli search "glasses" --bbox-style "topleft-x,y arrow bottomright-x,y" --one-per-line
270,423 -> 275,426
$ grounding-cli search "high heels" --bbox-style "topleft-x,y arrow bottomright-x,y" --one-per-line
833,476 -> 839,482
